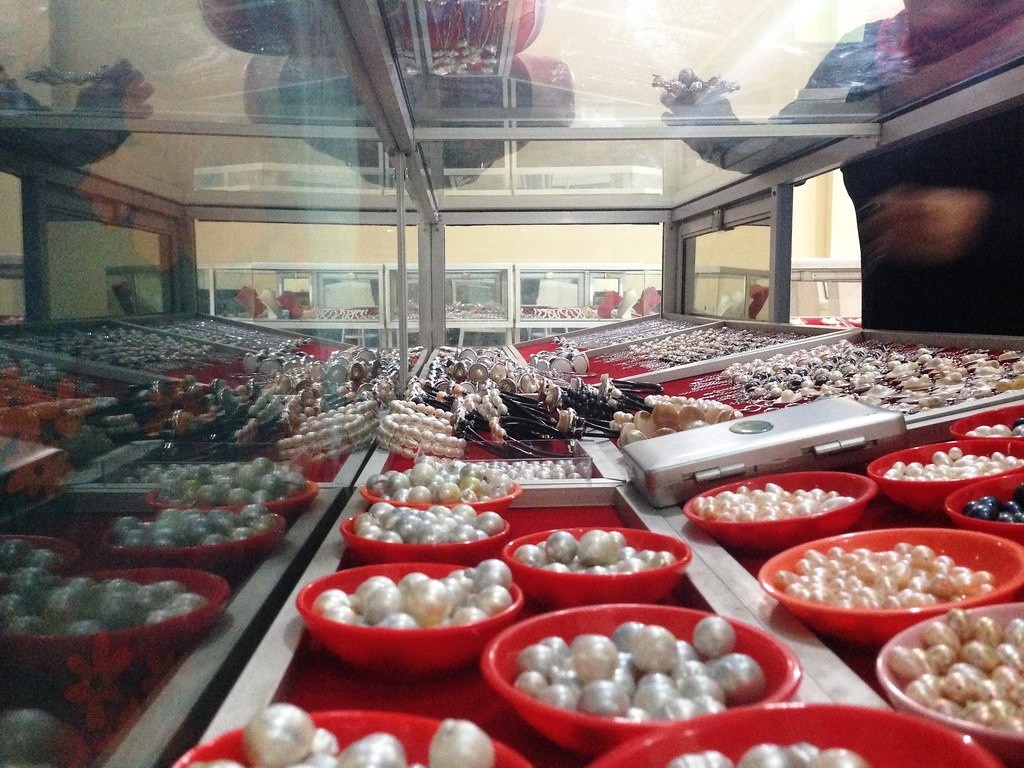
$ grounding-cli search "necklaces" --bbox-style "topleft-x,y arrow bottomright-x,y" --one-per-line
533,308 -> 586,319
565,318 -> 1024,414
384,0 -> 505,76
319,309 -> 368,320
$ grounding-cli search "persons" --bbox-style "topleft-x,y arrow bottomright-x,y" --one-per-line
661,0 -> 1024,334
0,58 -> 155,164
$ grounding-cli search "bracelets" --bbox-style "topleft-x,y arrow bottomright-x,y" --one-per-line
380,378 -> 663,458
90,384 -> 390,459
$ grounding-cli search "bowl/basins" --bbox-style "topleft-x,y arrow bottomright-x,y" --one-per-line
501,526 -> 702,635
295,561 -> 524,677
341,511 -> 511,569
865,405 -> 1024,546
757,527 -> 1024,648
359,480 -> 522,518
578,701 -> 1005,767
480,602 -> 804,755
0,458 -> 324,768
874,600 -> 1024,763
681,472 -> 880,551
169,709 -> 533,768
